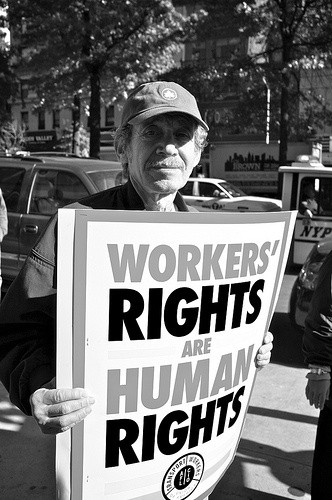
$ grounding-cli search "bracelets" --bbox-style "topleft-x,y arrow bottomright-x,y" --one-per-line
308,368 -> 330,375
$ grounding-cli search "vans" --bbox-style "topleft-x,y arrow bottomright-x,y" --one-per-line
0,152 -> 128,281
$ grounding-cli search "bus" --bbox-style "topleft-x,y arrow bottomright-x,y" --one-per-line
278,158 -> 332,266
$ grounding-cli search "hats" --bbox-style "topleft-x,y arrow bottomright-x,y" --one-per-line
121,81 -> 210,132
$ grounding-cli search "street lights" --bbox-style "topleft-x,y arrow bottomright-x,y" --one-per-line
261,75 -> 269,144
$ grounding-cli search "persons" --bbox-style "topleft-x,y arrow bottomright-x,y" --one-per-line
0,81 -> 274,434
304,246 -> 332,500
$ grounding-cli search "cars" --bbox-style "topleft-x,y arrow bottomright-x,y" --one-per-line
179,178 -> 282,215
